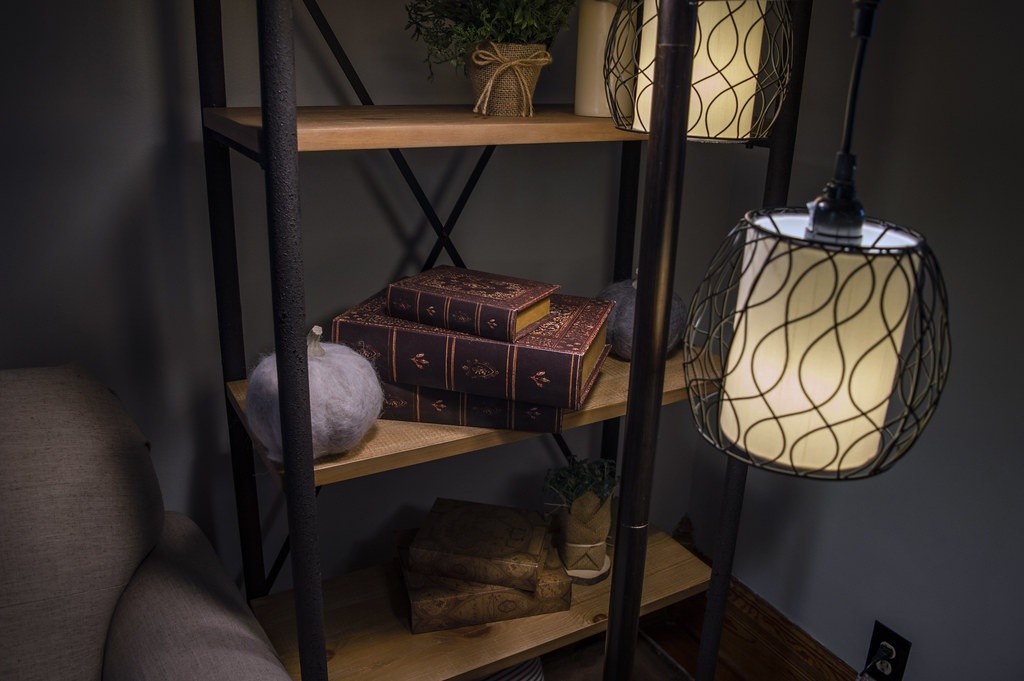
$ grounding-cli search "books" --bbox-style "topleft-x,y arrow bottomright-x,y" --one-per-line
324,264 -> 617,435
391,496 -> 575,633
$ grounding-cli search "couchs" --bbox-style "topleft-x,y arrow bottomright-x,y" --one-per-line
0,367 -> 291,681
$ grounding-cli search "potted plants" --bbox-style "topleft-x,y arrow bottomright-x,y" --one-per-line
542,454 -> 621,571
404,0 -> 577,117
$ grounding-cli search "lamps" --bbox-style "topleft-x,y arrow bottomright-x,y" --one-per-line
574,1 -> 638,117
683,0 -> 952,482
603,0 -> 795,144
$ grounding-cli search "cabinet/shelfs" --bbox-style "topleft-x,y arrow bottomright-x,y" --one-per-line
192,0 -> 815,681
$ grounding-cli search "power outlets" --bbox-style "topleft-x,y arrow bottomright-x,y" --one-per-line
864,619 -> 912,681
